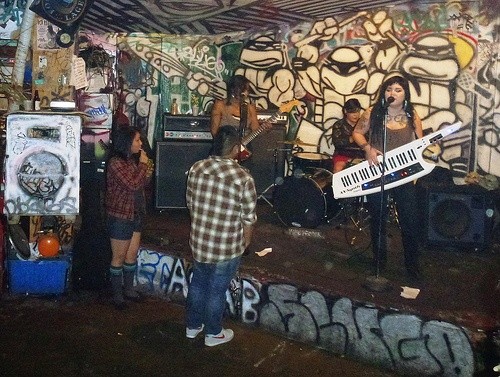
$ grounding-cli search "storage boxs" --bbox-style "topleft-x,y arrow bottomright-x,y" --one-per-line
7,254 -> 72,296
155,139 -> 213,208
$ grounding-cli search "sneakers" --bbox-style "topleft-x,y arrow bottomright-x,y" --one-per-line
186,323 -> 205,338
205,328 -> 235,346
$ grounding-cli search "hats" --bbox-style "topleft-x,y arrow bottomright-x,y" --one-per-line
344,98 -> 361,113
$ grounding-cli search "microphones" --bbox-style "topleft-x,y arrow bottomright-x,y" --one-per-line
379,96 -> 395,116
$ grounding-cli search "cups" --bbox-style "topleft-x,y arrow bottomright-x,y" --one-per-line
192,106 -> 199,116
23,100 -> 31,111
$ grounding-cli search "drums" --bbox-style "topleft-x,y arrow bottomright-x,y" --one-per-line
292,151 -> 330,175
273,167 -> 343,228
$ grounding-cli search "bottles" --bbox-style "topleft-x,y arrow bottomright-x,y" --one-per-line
171,99 -> 178,115
33,90 -> 41,111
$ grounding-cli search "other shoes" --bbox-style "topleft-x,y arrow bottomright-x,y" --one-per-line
111,298 -> 130,310
405,258 -> 420,277
371,253 -> 388,275
123,292 -> 144,300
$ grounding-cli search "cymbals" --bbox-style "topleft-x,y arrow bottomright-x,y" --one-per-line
277,140 -> 320,146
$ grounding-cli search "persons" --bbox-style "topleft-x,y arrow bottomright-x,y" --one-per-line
331,98 -> 369,175
350,75 -> 423,280
104,125 -> 155,317
184,125 -> 258,348
210,75 -> 274,172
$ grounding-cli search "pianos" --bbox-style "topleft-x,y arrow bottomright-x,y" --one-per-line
331,120 -> 463,199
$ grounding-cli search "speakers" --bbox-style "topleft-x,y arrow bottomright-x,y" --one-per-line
425,185 -> 489,250
155,141 -> 213,210
243,124 -> 287,203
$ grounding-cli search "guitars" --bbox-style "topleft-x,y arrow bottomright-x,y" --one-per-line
235,98 -> 299,163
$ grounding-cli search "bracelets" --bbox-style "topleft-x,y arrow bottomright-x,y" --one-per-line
360,141 -> 368,150
